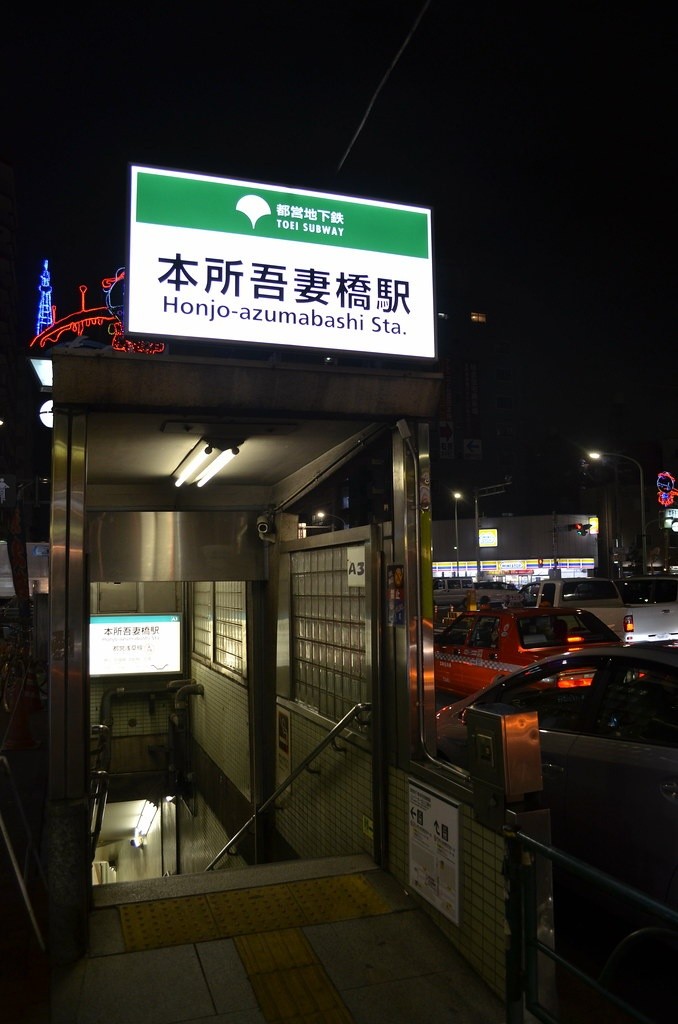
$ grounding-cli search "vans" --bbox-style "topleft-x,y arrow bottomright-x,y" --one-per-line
433,577 -> 473,606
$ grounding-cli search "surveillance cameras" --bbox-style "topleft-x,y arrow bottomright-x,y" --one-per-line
257,516 -> 268,533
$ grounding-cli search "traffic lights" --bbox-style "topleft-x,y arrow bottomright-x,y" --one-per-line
538,558 -> 543,567
576,523 -> 582,535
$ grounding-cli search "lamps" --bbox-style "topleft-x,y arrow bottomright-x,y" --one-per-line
169,436 -> 246,490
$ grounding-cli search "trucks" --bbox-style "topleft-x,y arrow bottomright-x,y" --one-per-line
669,566 -> 678,575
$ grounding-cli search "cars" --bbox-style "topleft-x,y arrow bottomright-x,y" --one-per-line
434,607 -> 624,700
437,645 -> 678,910
574,574 -> 678,648
517,578 -> 625,643
648,570 -> 656,575
466,581 -> 524,606
625,574 -> 633,579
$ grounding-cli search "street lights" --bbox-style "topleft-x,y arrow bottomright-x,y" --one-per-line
452,490 -> 463,580
644,517 -> 678,575
586,451 -> 648,573
